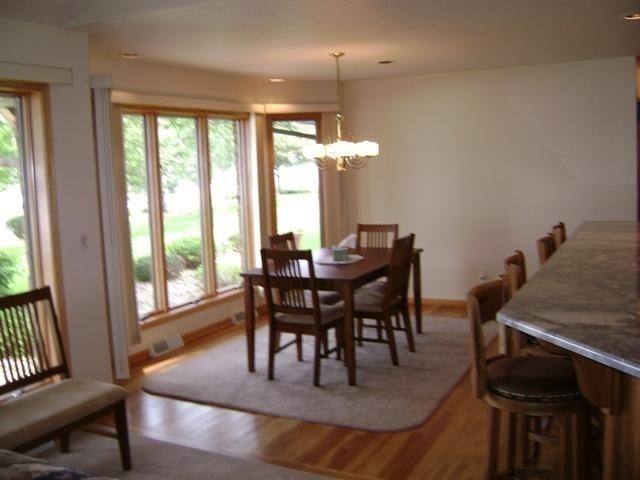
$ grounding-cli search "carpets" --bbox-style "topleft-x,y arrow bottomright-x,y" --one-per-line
26,428 -> 345,478
141,312 -> 500,432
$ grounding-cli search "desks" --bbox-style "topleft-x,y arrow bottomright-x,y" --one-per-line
238,247 -> 426,385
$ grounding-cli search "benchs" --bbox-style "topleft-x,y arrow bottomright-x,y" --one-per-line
2,285 -> 132,473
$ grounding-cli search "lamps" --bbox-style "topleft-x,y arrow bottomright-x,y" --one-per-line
302,50 -> 379,170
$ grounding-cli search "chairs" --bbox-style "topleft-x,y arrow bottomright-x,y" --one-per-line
551,220 -> 567,246
534,232 -> 555,264
465,280 -> 588,479
267,233 -> 341,356
354,223 -> 401,327
503,248 -> 572,461
355,235 -> 414,367
259,247 -> 346,387
